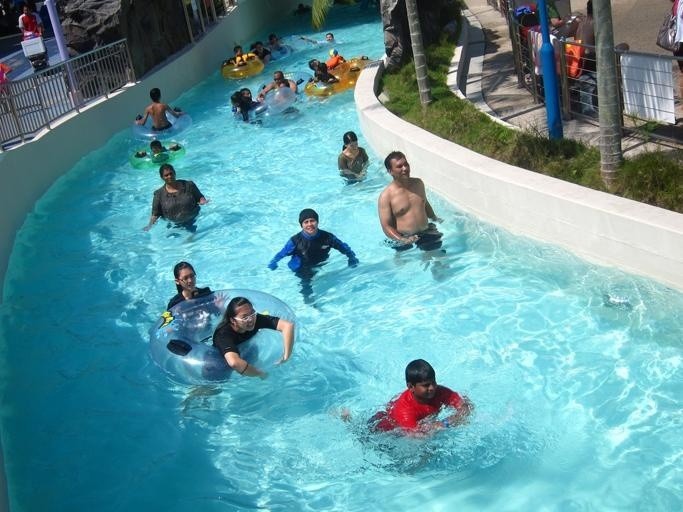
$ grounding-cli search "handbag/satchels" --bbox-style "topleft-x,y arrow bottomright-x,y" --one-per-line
655,2 -> 680,52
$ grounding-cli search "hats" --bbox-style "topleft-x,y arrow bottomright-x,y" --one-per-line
299,209 -> 319,224
329,48 -> 338,57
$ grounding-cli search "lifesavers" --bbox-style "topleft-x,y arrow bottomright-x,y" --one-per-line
222,56 -> 264,80
257,71 -> 313,113
305,56 -> 370,97
131,109 -> 192,141
129,141 -> 185,171
148,289 -> 294,385
251,44 -> 293,64
235,87 -> 297,123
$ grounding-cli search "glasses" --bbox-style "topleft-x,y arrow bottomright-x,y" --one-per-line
233,310 -> 257,322
179,274 -> 197,283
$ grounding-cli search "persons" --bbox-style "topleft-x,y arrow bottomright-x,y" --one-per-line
19,4 -> 44,38
167,261 -> 230,335
533,0 -> 683,137
338,131 -> 370,185
143,163 -> 206,241
341,358 -> 470,439
136,87 -> 187,159
268,208 -> 359,304
230,33 -> 346,123
378,151 -> 447,282
179,296 -> 295,411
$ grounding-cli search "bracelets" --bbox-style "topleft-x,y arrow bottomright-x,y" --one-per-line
442,418 -> 451,428
240,362 -> 250,375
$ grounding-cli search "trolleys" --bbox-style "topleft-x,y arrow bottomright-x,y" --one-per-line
21,32 -> 52,78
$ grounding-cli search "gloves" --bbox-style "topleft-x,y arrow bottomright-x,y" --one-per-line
348,257 -> 359,268
